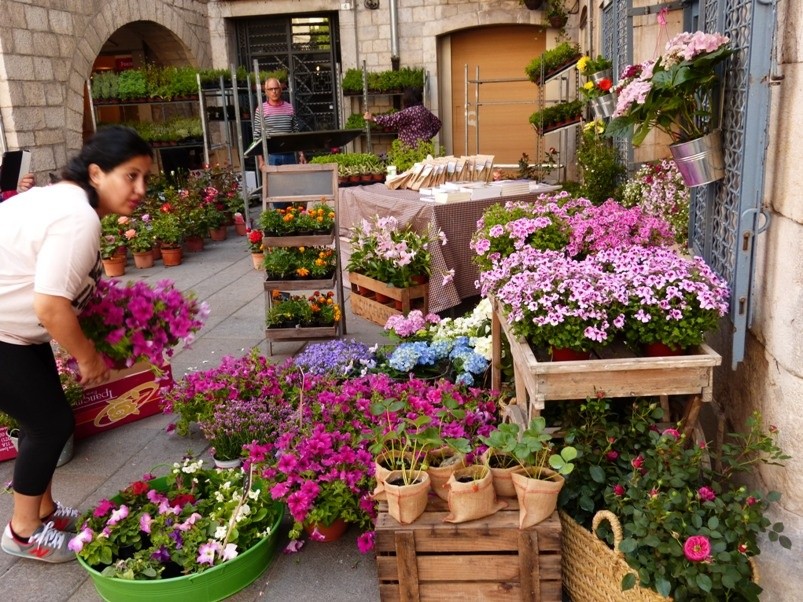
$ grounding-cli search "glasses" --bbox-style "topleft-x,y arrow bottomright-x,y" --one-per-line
266,87 -> 281,91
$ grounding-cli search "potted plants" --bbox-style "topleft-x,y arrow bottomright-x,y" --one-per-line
91,61 -> 426,146
524,0 -> 581,132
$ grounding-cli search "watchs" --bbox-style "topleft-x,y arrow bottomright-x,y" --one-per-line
298,153 -> 305,158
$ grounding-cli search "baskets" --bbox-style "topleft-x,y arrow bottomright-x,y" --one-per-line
557,509 -> 758,602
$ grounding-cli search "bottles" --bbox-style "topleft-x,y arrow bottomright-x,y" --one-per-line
387,166 -> 397,176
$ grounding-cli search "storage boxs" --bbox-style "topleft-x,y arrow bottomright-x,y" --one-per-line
0,355 -> 173,462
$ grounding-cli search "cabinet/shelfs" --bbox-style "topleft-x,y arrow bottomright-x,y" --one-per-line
340,64 -> 430,135
83,74 -> 233,180
464,54 -> 583,183
260,162 -> 346,356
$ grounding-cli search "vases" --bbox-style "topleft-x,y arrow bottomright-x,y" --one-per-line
638,340 -> 687,356
186,237 -> 205,252
251,253 -> 266,270
359,286 -> 374,297
375,292 -> 391,304
161,247 -> 182,265
411,276 -> 426,286
103,254 -> 126,277
550,343 -> 591,361
208,226 -> 227,241
133,249 -> 155,268
397,300 -> 414,311
590,68 -> 724,188
234,218 -> 254,236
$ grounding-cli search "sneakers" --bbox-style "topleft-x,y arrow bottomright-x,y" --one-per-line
42,501 -> 85,533
1,521 -> 80,562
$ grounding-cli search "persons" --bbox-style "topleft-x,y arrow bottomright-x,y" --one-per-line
0,125 -> 153,563
253,77 -> 307,211
0,174 -> 36,204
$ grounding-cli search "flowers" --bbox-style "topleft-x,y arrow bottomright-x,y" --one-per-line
56,159 -> 793,601
576,29 -> 733,148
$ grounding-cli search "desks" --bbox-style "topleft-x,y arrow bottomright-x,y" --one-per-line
337,178 -> 559,312
